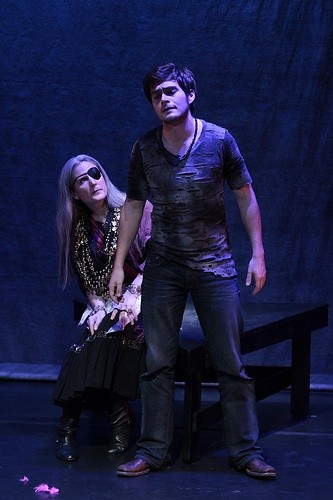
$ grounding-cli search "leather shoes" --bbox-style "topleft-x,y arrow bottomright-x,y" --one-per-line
116,459 -> 151,476
245,457 -> 276,477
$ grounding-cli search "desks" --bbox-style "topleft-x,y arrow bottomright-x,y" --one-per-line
66,300 -> 330,464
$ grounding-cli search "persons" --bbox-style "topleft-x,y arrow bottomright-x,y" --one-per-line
51,155 -> 153,464
108,64 -> 276,477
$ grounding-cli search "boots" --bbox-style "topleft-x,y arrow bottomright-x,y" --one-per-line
56,402 -> 76,461
105,391 -> 137,456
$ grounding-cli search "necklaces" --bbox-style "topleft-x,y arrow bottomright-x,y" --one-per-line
158,118 -> 198,161
